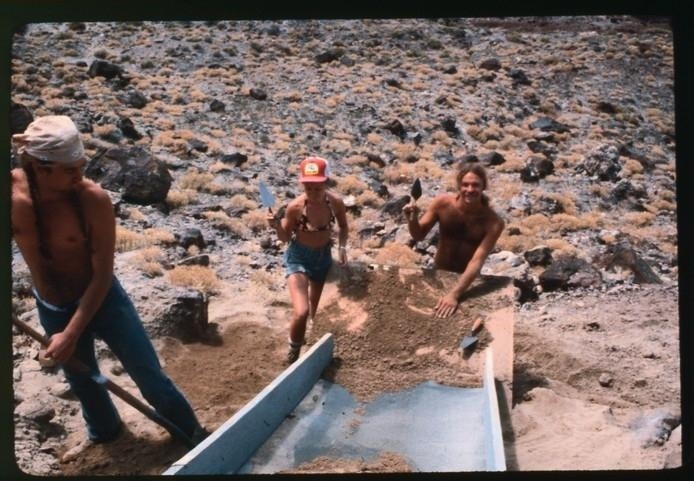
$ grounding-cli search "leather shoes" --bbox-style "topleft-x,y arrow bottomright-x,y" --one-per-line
60,419 -> 128,463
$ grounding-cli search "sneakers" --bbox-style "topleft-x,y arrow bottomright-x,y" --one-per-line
286,339 -> 306,364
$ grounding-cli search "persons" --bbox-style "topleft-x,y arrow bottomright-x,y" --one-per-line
402,165 -> 505,319
266,158 -> 348,364
10,116 -> 208,447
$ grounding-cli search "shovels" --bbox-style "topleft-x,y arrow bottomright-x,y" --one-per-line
460,317 -> 482,349
411,178 -> 422,222
259,179 -> 276,212
13,316 -> 212,448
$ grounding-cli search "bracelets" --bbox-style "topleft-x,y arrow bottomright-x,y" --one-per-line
339,246 -> 345,250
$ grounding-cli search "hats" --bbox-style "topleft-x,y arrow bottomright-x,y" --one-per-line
298,155 -> 330,183
